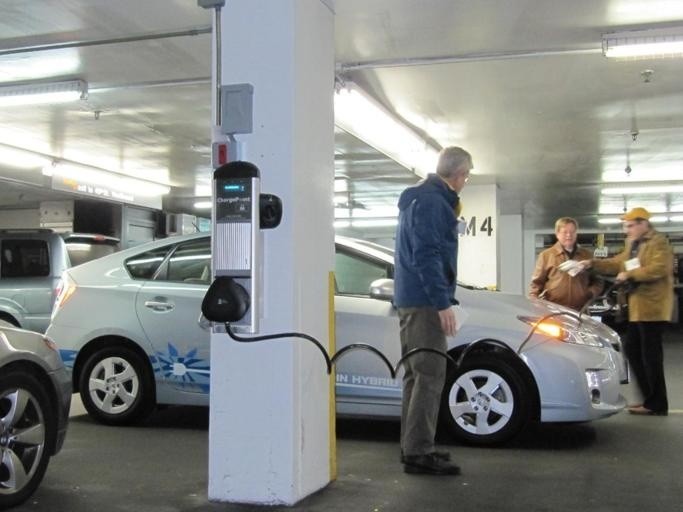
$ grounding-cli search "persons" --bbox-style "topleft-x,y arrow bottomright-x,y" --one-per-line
579,207 -> 673,417
392,146 -> 474,476
529,217 -> 604,315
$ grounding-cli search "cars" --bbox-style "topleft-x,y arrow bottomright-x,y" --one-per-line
47,232 -> 630,448
0,319 -> 71,510
0,228 -> 121,334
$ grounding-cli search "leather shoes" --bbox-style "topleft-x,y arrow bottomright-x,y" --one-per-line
432,448 -> 451,460
627,405 -> 668,415
402,455 -> 461,476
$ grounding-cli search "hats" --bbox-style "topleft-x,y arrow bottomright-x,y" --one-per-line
622,207 -> 654,221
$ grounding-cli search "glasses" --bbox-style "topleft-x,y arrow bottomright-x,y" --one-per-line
464,175 -> 471,185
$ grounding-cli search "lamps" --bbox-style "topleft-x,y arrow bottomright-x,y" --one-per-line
0,80 -> 88,109
602,27 -> 683,58
